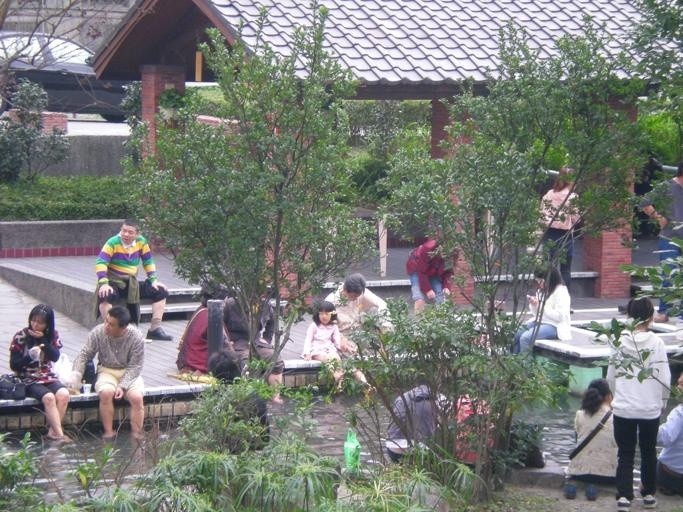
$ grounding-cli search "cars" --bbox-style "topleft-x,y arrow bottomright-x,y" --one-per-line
1,30 -> 140,121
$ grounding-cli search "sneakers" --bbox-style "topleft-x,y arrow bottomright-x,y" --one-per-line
586,487 -> 597,500
644,496 -> 658,508
563,485 -> 578,500
616,496 -> 631,512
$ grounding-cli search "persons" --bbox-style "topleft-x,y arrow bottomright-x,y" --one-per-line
302,274 -> 393,397
385,375 -> 497,471
406,237 -> 456,313
96,219 -> 173,341
70,306 -> 144,435
538,167 -> 581,313
607,296 -> 672,511
567,377 -> 620,485
656,374 -> 683,497
10,304 -> 69,440
635,164 -> 683,323
512,264 -> 571,356
178,281 -> 285,405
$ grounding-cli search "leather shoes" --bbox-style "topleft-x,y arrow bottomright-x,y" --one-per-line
146,326 -> 173,341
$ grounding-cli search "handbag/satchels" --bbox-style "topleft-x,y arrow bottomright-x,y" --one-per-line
0,374 -> 26,401
81,360 -> 96,394
